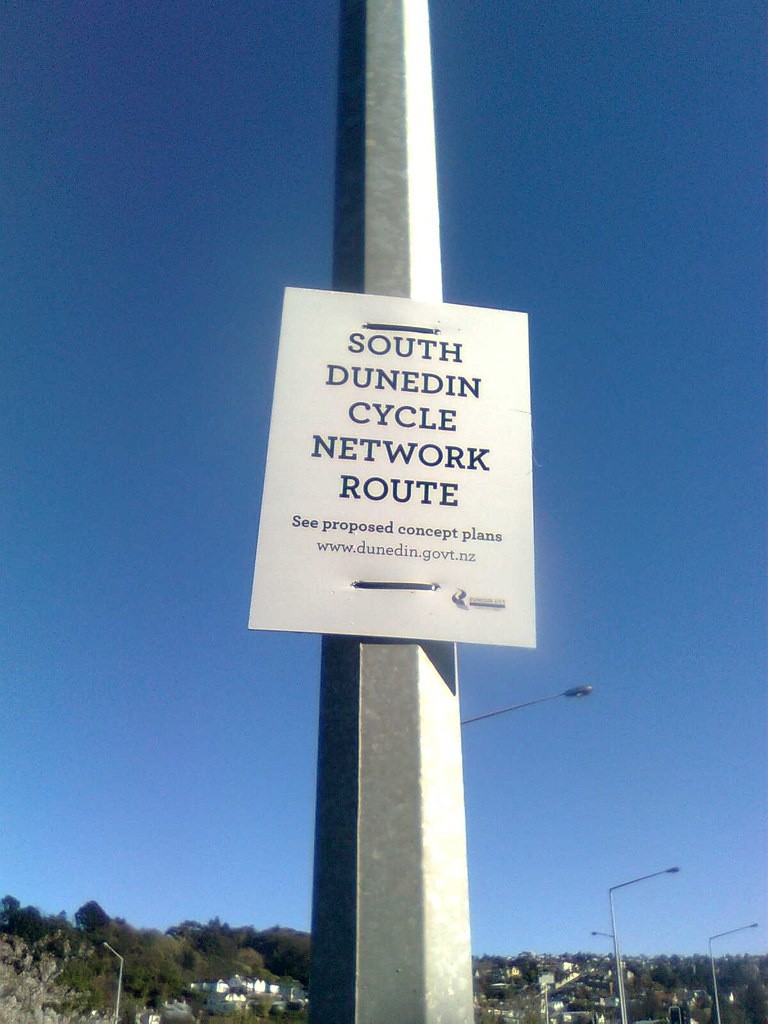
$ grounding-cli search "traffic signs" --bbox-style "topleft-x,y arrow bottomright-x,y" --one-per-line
253,287 -> 539,651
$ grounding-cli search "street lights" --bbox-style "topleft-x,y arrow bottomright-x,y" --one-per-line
592,868 -> 681,1024
708,923 -> 759,1024
102,942 -> 124,1024
